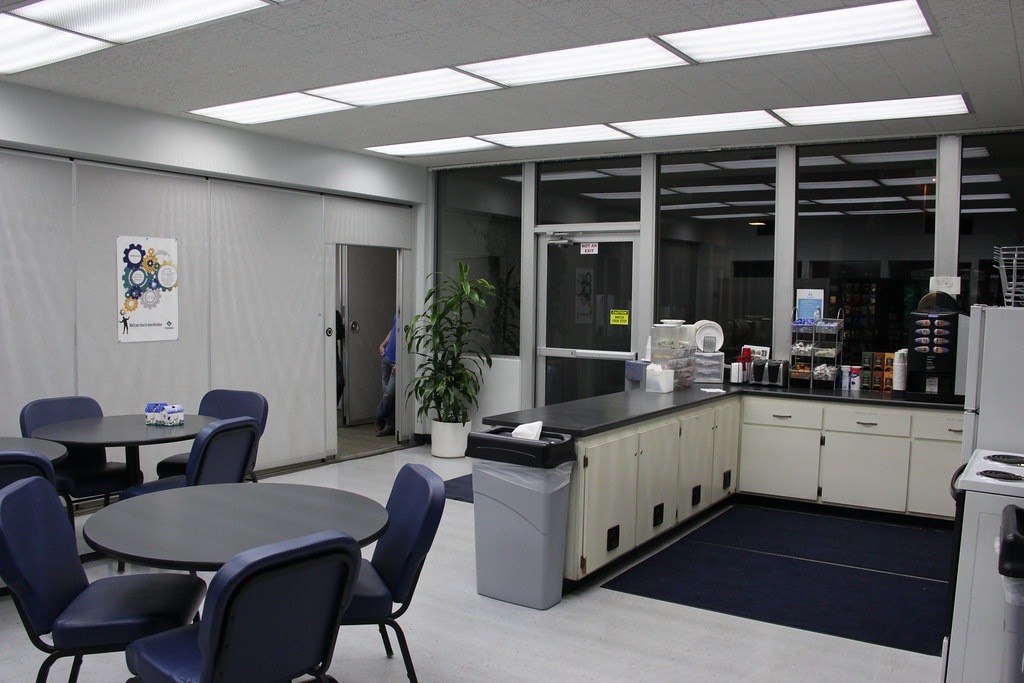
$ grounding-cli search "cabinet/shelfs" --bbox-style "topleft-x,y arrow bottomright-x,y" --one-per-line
945,491 -> 1024,683
787,306 -> 845,391
736,397 -> 963,519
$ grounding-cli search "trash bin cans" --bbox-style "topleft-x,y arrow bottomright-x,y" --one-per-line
464,425 -> 577,610
996,505 -> 1024,683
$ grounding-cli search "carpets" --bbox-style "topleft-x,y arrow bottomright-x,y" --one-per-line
600,497 -> 961,659
443,473 -> 473,505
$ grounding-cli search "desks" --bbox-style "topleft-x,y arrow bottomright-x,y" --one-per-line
82,483 -> 389,626
563,398 -> 741,581
0,436 -> 75,532
31,414 -> 222,487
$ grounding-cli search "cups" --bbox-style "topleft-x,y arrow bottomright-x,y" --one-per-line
892,348 -> 908,397
731,347 -> 780,386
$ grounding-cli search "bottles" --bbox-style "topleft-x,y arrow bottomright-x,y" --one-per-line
828,269 -> 899,394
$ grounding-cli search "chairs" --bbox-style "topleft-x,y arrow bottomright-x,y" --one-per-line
21,396 -> 144,526
125,529 -> 361,683
338,462 -> 445,683
0,476 -> 207,683
117,415 -> 259,574
157,388 -> 269,484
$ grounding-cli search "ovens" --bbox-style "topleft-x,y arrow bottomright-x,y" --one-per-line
940,462 -> 1024,683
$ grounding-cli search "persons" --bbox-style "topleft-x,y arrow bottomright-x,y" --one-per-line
375,314 -> 396,437
336,310 -> 345,406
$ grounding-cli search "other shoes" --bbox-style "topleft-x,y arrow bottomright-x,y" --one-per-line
376,419 -> 394,436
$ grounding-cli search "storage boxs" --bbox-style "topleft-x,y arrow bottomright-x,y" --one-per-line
695,352 -> 724,383
646,367 -> 673,394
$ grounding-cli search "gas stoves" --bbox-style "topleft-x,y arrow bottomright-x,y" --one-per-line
950,449 -> 1024,499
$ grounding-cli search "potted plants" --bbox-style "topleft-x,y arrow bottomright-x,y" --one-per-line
400,260 -> 498,458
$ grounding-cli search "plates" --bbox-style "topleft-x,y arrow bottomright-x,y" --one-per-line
653,318 -> 685,327
694,320 -> 723,353
